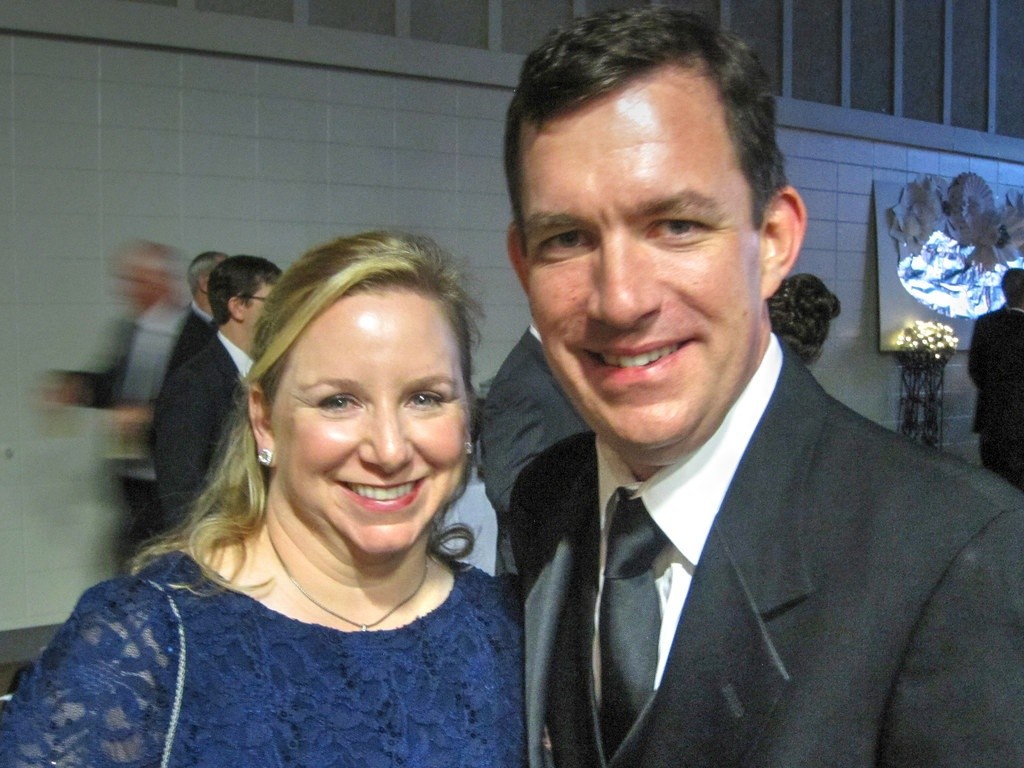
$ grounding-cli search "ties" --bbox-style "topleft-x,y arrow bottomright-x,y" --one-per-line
599,487 -> 667,764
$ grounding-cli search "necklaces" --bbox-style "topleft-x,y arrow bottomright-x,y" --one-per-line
268,525 -> 428,632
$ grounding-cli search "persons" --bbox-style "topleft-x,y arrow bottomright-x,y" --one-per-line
765,273 -> 842,366
0,228 -> 526,767
501,0 -> 1024,768
31,240 -> 284,578
966,269 -> 1024,494
479,321 -> 590,577
